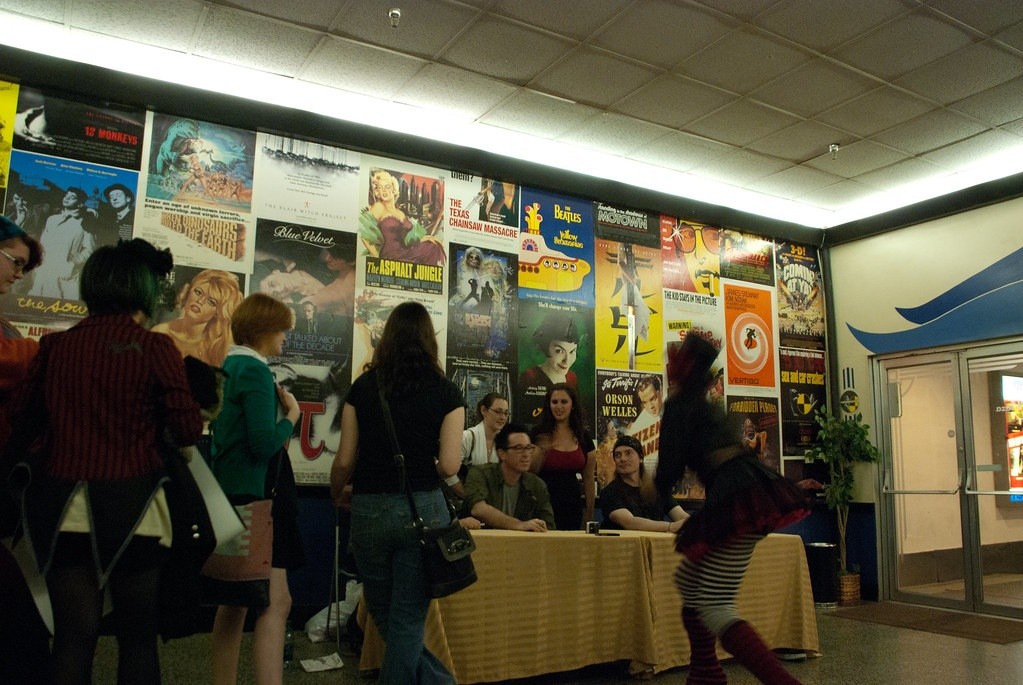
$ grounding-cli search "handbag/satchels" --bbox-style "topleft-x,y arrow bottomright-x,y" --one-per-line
418,516 -> 478,599
200,499 -> 276,581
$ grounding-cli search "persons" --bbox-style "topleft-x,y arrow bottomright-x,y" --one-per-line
0,217 -> 203,685
151,271 -> 243,368
268,229 -> 355,336
638,374 -> 663,417
6,182 -> 135,300
741,421 -> 767,457
597,417 -> 617,493
599,435 -> 691,532
528,382 -> 596,530
435,392 -> 509,501
209,294 -> 301,685
460,421 -> 556,532
653,332 -> 816,685
356,169 -> 580,423
330,300 -> 468,685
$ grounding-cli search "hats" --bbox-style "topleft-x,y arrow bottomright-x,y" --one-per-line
612,435 -> 644,458
666,334 -> 719,393
103,183 -> 135,206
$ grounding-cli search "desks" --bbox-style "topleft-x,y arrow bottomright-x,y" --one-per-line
356,529 -> 824,684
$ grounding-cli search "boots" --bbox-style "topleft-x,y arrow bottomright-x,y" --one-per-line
720,619 -> 803,685
680,607 -> 728,685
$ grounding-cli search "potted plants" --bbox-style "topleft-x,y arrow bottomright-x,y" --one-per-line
798,398 -> 879,605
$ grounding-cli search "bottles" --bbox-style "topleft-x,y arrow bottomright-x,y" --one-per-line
283,620 -> 294,670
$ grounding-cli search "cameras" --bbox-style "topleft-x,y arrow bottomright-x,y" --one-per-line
586,520 -> 599,534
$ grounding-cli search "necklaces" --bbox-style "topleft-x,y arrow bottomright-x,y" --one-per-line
487,440 -> 494,453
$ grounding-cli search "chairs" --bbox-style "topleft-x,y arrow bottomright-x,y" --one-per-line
325,484 -> 361,658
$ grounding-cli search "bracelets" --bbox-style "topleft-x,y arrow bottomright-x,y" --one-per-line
668,521 -> 672,531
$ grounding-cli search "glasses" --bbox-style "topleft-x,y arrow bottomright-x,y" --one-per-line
505,443 -> 537,453
0,249 -> 30,274
488,407 -> 510,416
